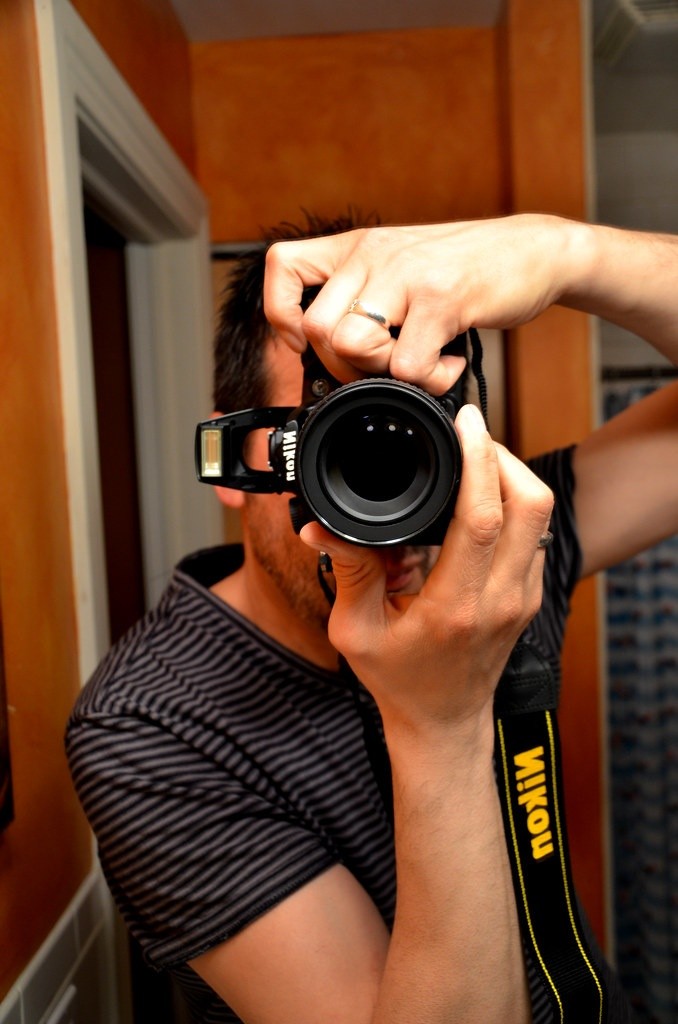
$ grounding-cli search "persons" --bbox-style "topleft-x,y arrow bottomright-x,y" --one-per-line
60,206 -> 678,1024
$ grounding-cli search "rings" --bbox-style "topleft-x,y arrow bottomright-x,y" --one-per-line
345,297 -> 392,330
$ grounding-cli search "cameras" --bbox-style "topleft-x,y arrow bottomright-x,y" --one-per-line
193,284 -> 463,545
